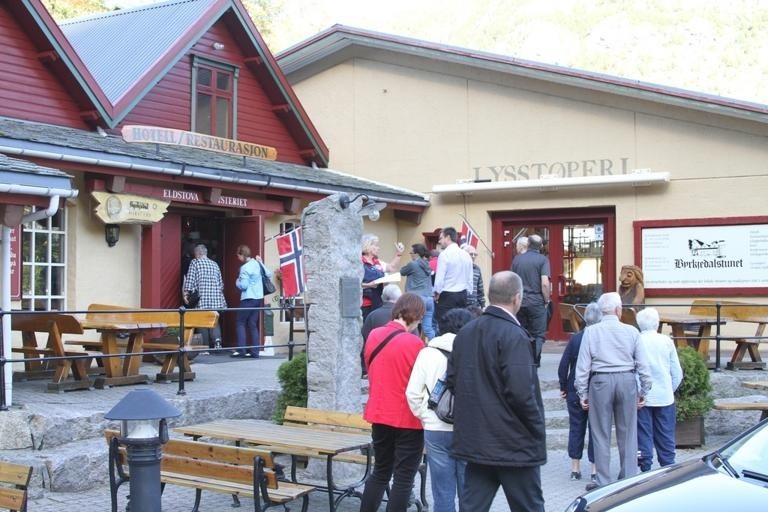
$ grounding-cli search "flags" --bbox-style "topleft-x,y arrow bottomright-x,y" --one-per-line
275,227 -> 307,299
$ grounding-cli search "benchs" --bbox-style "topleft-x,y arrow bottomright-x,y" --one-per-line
64,301 -> 222,383
0,458 -> 34,511
10,309 -> 92,394
104,428 -> 317,511
688,297 -> 768,373
273,405 -> 429,508
555,300 -> 641,338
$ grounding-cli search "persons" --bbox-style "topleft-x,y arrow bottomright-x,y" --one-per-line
182,243 -> 228,355
229,244 -> 272,358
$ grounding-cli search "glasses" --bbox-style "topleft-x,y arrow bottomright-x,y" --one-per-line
469,251 -> 480,257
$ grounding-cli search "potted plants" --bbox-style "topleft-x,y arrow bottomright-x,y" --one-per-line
670,346 -> 716,446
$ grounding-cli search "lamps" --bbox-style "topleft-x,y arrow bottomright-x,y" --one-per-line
105,223 -> 120,247
339,191 -> 387,223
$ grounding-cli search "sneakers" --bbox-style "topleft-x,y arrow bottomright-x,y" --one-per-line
200,341 -> 260,361
568,470 -> 609,491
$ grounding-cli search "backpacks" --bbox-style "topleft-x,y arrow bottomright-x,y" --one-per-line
420,343 -> 456,426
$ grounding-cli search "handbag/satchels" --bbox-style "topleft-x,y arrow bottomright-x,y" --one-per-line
182,289 -> 200,309
545,299 -> 555,324
260,273 -> 278,296
361,265 -> 385,303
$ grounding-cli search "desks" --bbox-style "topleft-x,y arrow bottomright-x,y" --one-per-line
741,378 -> 768,393
75,318 -> 169,390
172,417 -> 423,511
710,393 -> 767,423
656,315 -> 728,371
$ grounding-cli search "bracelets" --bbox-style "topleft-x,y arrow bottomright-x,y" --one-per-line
184,294 -> 191,297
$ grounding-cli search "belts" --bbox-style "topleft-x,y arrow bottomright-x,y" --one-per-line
591,370 -> 635,376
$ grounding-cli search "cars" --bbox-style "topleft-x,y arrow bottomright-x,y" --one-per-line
563,417 -> 767,512
